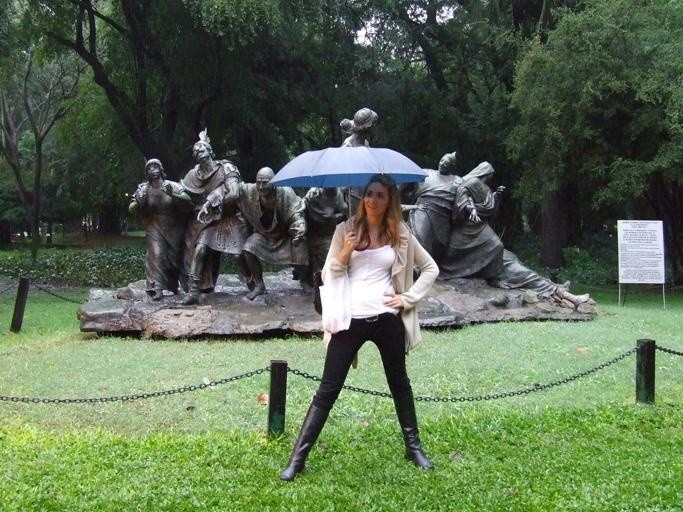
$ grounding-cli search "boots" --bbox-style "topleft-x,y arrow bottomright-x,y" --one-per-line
279,403 -> 329,481
394,405 -> 435,470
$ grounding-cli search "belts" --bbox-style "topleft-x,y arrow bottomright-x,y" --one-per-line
350,312 -> 399,323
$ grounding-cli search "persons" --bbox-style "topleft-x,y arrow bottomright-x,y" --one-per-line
400,203 -> 428,211
502,249 -> 590,306
340,107 -> 379,215
279,175 -> 434,482
303,186 -> 349,286
436,161 -> 511,290
407,152 -> 481,281
128,158 -> 192,300
178,140 -> 256,306
196,167 -> 316,301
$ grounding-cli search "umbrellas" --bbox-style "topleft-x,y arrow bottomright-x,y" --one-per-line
267,147 -> 428,251
